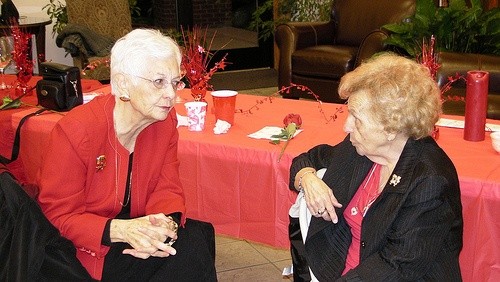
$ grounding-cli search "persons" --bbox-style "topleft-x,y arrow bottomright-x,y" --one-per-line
290,51 -> 464,282
37,29 -> 219,282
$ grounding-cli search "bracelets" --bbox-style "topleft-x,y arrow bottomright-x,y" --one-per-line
299,171 -> 317,193
164,216 -> 178,246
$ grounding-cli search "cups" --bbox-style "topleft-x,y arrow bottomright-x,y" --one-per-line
184,101 -> 206,132
490,130 -> 500,153
211,90 -> 238,127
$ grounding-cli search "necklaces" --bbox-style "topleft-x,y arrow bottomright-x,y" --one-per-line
115,114 -> 136,207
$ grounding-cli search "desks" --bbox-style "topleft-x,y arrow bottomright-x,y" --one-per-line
0,16 -> 52,75
13,86 -> 500,282
0,75 -> 103,197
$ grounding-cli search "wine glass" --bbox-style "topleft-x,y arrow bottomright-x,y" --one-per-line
0,37 -> 11,90
175,46 -> 187,103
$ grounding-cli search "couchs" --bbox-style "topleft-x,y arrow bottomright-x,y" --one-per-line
275,0 -> 500,120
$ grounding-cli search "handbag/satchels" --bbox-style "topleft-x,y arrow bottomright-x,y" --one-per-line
35,62 -> 83,113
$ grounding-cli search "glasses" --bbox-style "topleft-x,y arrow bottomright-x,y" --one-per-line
133,76 -> 187,91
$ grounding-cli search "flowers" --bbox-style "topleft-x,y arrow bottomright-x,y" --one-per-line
270,114 -> 302,160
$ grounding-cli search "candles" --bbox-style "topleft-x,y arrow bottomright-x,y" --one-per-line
463,70 -> 489,142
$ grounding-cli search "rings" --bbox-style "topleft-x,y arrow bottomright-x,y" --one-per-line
314,213 -> 319,216
318,207 -> 326,215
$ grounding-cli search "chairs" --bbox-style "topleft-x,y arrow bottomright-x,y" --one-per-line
62,0 -> 136,82
0,170 -> 217,282
